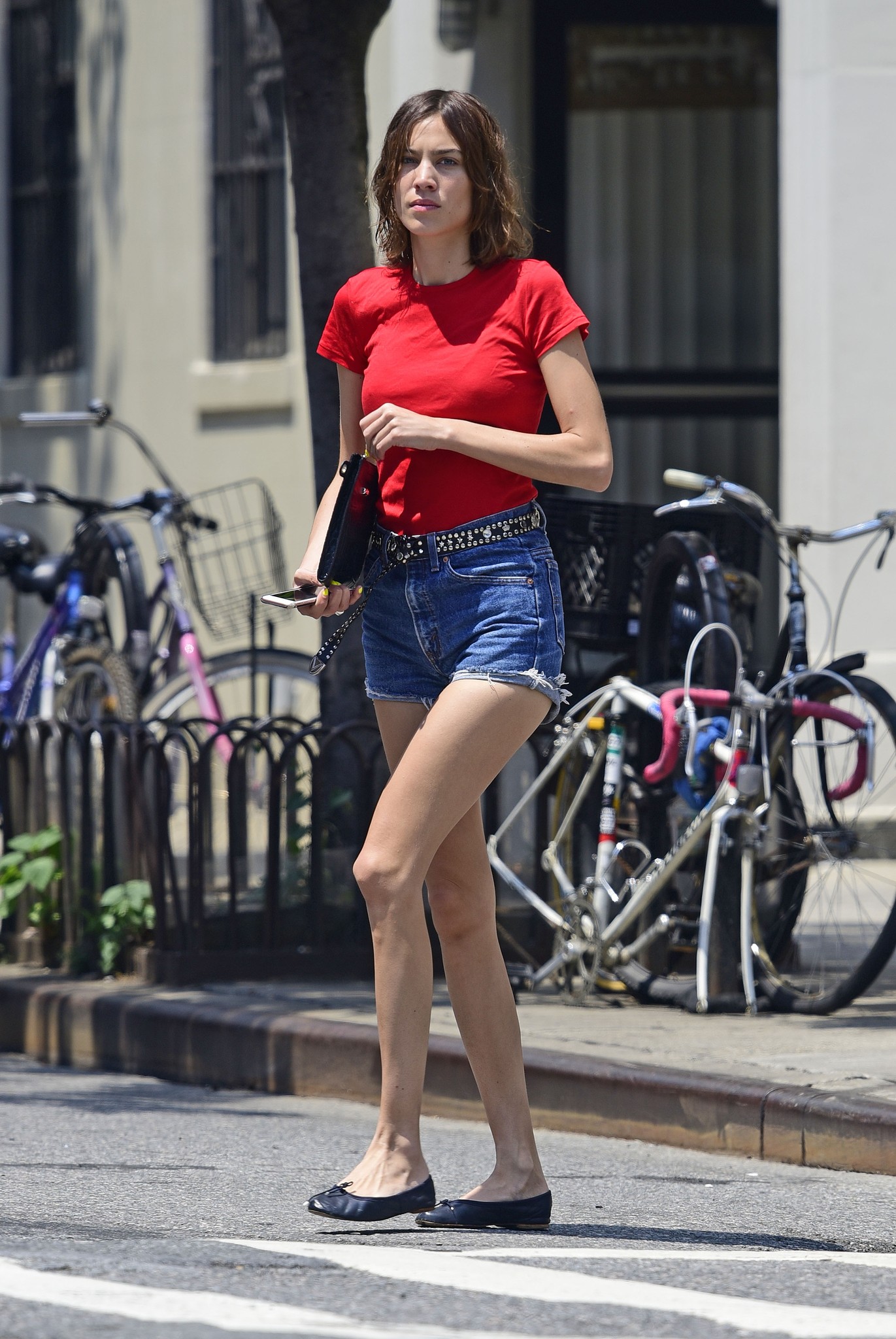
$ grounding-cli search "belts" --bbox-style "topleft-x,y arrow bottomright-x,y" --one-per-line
366,514 -> 541,572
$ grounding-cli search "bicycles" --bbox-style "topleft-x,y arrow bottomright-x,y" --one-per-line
0,399 -> 316,925
508,468 -> 896,1014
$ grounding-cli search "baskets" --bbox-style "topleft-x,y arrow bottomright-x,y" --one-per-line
175,476 -> 294,637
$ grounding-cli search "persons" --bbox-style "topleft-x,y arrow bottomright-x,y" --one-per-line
293,88 -> 613,1227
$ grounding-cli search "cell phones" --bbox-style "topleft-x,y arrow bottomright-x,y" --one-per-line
261,585 -> 316,610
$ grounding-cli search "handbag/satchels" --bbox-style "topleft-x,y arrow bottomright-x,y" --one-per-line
312,453 -> 382,587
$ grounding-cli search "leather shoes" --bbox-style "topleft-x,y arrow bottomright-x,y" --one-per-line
414,1189 -> 553,1232
308,1174 -> 437,1223
